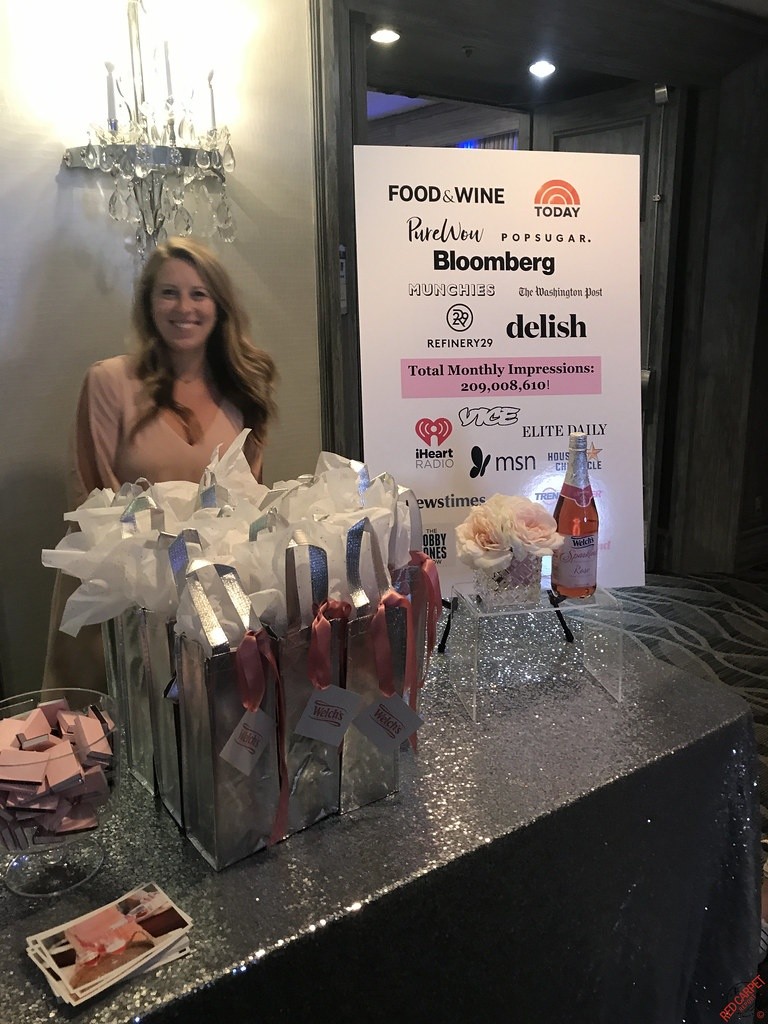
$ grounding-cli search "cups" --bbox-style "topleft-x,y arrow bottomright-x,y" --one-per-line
0,687 -> 120,897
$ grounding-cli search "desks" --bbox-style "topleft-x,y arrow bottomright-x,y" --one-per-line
0,610 -> 762,1024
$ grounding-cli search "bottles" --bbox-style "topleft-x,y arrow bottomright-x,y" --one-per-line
551,432 -> 599,599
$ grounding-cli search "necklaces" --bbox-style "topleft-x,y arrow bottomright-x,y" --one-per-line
177,367 -> 206,384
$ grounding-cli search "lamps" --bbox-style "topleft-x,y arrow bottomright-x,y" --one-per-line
68,5 -> 235,251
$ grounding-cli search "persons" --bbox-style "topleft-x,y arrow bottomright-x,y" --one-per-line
40,236 -> 276,711
